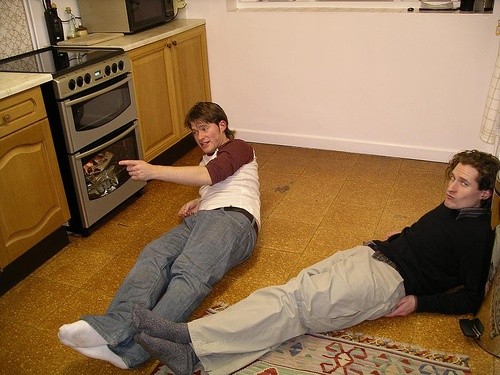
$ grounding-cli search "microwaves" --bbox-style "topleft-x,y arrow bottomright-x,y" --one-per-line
78,0 -> 174,35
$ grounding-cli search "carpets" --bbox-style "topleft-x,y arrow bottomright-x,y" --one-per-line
140,300 -> 472,375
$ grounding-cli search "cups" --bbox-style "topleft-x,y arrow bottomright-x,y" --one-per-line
75,27 -> 88,37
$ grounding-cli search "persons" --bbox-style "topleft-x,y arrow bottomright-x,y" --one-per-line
131,148 -> 499,375
55,101 -> 263,371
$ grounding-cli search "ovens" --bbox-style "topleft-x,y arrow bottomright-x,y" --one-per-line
40,51 -> 148,230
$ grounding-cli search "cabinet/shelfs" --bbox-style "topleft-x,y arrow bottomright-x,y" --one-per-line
125,24 -> 212,162
0,85 -> 73,275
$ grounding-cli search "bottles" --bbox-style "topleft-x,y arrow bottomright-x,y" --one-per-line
65,7 -> 77,40
44,8 -> 65,45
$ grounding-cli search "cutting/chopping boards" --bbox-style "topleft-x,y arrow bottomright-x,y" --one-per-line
57,32 -> 124,46
164,0 -> 187,22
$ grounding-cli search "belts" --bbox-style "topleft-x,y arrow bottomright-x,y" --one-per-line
213,207 -> 258,237
